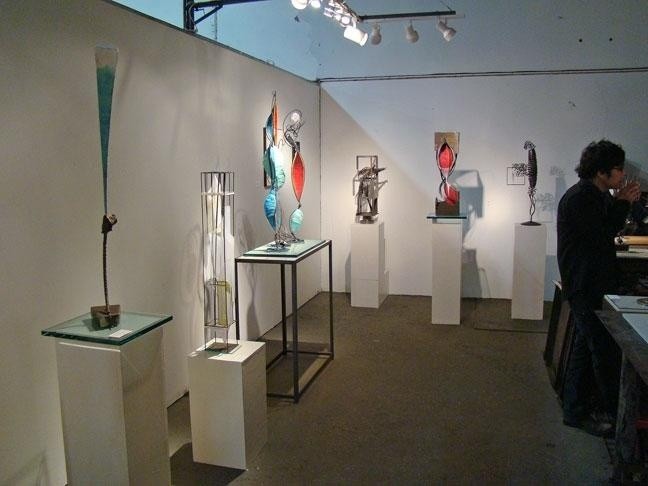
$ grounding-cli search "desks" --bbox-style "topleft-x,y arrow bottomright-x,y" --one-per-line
234,240 -> 335,402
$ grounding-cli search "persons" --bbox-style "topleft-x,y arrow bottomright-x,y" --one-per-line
557,139 -> 641,435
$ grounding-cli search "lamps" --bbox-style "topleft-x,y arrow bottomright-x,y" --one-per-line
344,12 -> 464,47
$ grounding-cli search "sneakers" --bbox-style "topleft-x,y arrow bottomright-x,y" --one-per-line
563,412 -> 615,437
